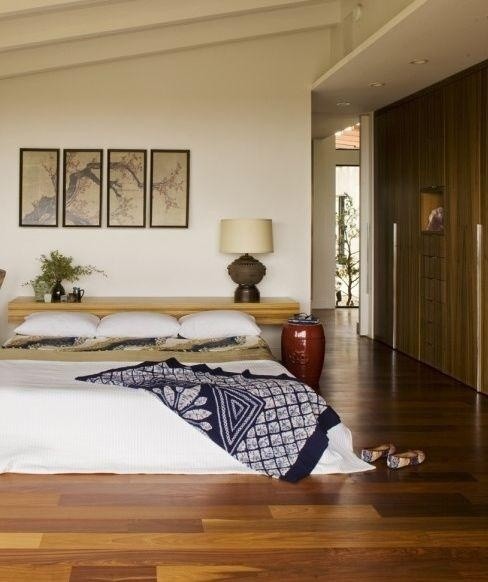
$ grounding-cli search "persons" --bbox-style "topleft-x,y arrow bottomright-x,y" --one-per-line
427,204 -> 445,232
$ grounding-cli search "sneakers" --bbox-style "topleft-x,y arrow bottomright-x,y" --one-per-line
361,443 -> 396,463
387,449 -> 426,470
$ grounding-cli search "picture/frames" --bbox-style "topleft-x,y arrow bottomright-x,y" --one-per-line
151,149 -> 190,228
19,149 -> 59,226
106,148 -> 146,227
63,148 -> 101,228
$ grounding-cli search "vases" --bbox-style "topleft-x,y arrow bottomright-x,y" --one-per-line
30,282 -> 53,301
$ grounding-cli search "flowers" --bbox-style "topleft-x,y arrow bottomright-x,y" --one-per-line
20,249 -> 108,286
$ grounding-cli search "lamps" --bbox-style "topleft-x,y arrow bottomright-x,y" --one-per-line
218,218 -> 274,301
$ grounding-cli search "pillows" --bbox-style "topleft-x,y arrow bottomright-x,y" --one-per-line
177,309 -> 261,340
16,311 -> 94,339
94,312 -> 179,340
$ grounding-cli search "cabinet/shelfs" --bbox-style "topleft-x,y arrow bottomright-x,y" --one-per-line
419,89 -> 444,185
420,234 -> 445,369
447,74 -> 485,395
372,97 -> 418,357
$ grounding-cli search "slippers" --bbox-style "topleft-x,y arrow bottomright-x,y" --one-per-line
288,313 -> 308,323
300,314 -> 320,323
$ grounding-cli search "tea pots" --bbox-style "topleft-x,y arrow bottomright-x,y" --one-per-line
73,286 -> 84,303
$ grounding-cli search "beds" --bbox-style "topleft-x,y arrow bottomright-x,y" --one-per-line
0,308 -> 315,469
